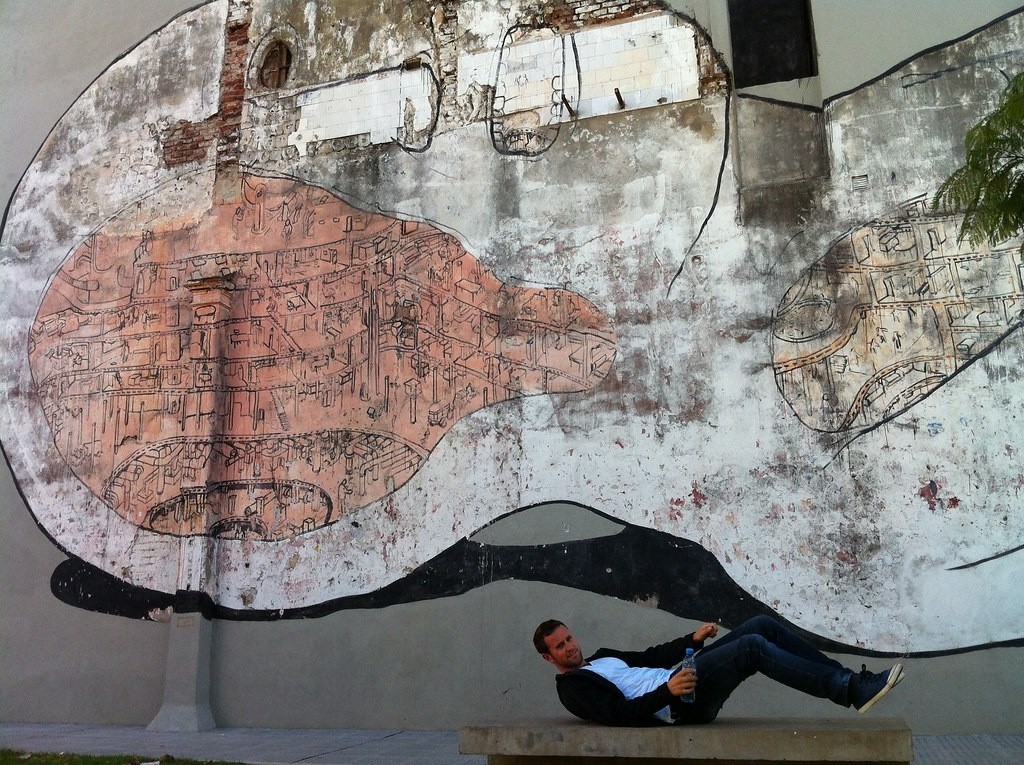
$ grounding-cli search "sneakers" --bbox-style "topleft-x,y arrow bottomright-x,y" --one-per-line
849,663 -> 905,714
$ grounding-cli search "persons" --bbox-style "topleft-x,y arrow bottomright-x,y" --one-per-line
533,614 -> 910,727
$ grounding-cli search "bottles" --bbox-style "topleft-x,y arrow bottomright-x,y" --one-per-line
680,648 -> 696,703
